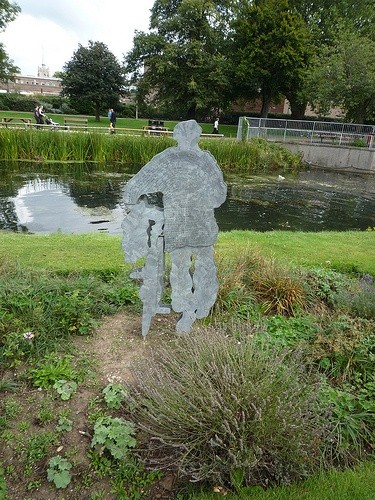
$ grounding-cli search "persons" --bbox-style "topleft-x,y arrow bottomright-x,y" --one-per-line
212,117 -> 219,137
38,105 -> 47,129
34,104 -> 41,129
121,119 -> 228,338
109,108 -> 116,134
49,118 -> 55,124
108,109 -> 112,121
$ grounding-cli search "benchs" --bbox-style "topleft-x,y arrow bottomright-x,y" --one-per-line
63,118 -> 87,130
4,117 -> 31,127
143,125 -> 168,135
320,133 -> 337,142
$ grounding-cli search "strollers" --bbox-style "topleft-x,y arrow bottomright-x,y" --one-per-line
44,115 -> 60,131
